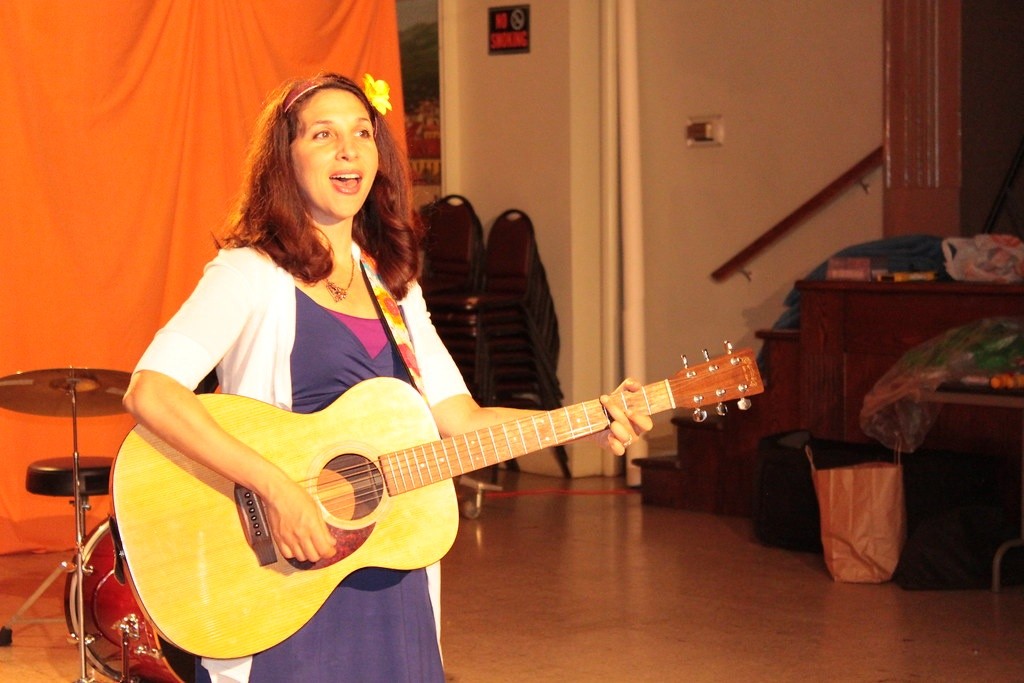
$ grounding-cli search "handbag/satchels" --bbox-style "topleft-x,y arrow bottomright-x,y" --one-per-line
805,433 -> 908,585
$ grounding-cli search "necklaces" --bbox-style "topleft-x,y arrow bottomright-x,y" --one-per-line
322,255 -> 355,302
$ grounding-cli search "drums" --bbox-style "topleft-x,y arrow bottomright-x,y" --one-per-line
61,515 -> 195,683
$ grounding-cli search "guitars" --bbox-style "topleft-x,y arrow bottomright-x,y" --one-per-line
106,340 -> 766,661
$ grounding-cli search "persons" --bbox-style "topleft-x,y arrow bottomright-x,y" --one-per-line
121,69 -> 654,683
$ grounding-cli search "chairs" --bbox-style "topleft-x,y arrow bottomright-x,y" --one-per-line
418,192 -> 572,488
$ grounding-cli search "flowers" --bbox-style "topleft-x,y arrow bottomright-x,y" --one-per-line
363,71 -> 396,115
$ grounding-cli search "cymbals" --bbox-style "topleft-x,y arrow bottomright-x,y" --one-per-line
0,367 -> 132,417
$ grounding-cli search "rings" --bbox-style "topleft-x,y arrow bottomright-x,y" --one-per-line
623,434 -> 632,446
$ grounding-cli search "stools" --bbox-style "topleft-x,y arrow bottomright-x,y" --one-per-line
25,456 -> 113,550
